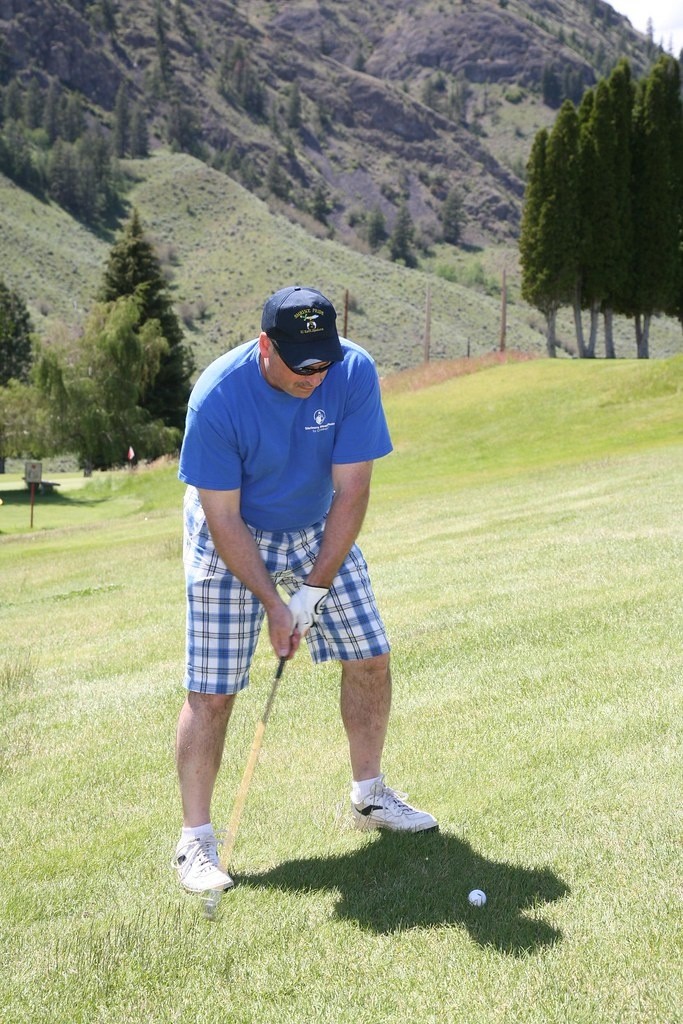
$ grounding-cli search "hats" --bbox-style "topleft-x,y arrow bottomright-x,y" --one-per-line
261,286 -> 343,369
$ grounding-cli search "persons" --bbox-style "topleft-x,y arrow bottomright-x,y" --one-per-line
170,287 -> 439,894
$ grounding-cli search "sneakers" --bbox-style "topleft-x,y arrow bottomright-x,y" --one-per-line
350,776 -> 439,834
170,829 -> 234,893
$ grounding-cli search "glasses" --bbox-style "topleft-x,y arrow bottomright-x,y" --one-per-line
268,336 -> 336,376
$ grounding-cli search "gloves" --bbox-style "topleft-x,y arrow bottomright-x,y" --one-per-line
286,583 -> 330,637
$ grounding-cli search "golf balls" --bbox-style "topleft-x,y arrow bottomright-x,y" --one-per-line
469,888 -> 487,907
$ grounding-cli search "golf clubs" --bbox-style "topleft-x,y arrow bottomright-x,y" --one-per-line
201,657 -> 284,918
